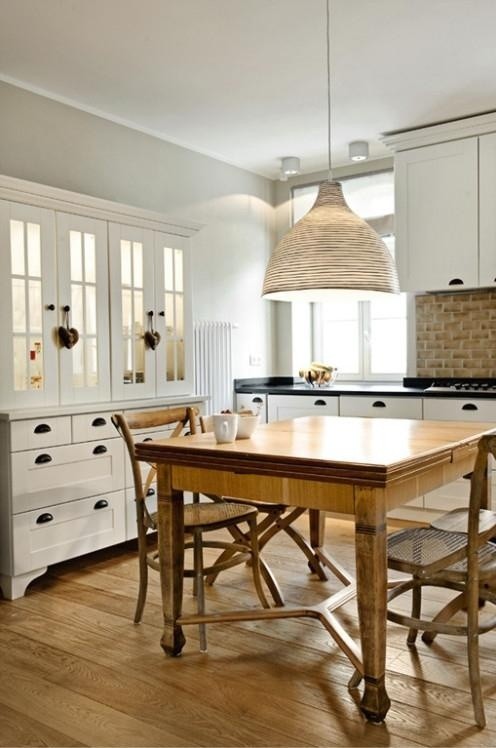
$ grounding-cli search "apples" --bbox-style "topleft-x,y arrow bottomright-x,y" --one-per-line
305,371 -> 332,386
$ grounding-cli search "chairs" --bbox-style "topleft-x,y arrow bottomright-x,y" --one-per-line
364,432 -> 494,733
419,499 -> 493,650
197,408 -> 333,605
107,402 -> 274,654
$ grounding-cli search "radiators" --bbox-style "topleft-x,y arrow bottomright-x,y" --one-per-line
187,317 -> 237,410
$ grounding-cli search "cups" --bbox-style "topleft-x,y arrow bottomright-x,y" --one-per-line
234,414 -> 260,439
123,369 -> 144,384
211,413 -> 238,443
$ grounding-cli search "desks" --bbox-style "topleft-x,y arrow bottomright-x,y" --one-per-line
128,409 -> 496,726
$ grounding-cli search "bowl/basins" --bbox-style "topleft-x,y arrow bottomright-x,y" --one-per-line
299,369 -> 339,388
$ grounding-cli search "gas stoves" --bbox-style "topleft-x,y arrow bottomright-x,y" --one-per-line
423,380 -> 496,393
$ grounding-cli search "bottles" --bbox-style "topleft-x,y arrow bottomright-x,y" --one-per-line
27,340 -> 43,391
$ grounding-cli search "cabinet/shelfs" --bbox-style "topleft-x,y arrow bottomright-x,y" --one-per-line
264,389 -> 340,416
478,106 -> 496,297
106,197 -> 207,410
1,171 -> 111,417
337,392 -> 422,513
0,401 -> 126,604
414,396 -> 496,530
378,116 -> 483,297
123,397 -> 213,542
236,387 -> 267,414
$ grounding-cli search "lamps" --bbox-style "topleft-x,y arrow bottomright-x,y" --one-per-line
278,155 -> 301,180
347,139 -> 372,163
259,2 -> 405,309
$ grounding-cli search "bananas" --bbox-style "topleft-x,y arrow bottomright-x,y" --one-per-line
310,363 -> 336,374
